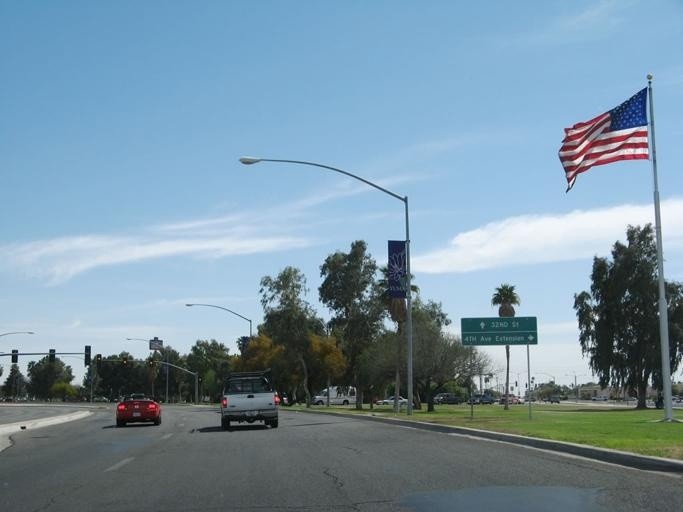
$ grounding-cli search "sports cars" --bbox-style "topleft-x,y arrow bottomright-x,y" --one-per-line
115,394 -> 161,425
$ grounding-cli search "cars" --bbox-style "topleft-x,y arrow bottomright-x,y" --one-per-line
467,394 -> 493,404
671,393 -> 680,406
88,395 -> 110,403
591,395 -> 608,401
498,394 -> 561,404
238,157 -> 414,416
376,394 -> 413,405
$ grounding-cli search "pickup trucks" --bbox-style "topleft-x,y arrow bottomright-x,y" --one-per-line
432,393 -> 463,405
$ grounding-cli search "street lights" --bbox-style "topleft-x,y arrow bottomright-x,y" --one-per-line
564,373 -> 586,404
127,337 -> 169,402
0,331 -> 34,339
183,302 -> 253,372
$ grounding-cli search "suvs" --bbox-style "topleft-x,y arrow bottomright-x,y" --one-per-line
219,370 -> 278,430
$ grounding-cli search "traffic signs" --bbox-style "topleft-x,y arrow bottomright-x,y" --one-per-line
461,332 -> 537,345
460,317 -> 536,331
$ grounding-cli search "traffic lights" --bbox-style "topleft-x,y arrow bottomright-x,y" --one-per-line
122,357 -> 127,368
148,357 -> 153,368
197,377 -> 201,385
96,354 -> 101,368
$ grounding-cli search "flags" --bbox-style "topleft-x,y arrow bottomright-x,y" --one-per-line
386,239 -> 408,299
557,89 -> 650,193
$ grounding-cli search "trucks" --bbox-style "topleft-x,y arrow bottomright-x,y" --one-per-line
310,385 -> 357,406
